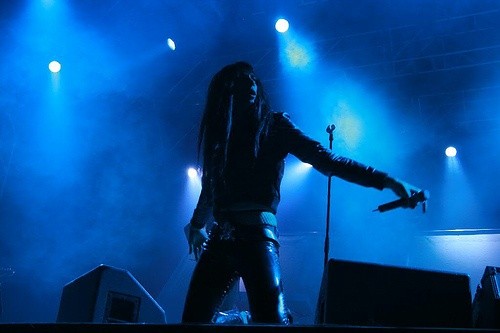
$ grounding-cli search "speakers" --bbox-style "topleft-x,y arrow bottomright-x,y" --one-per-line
56,264 -> 166,324
472,266 -> 500,329
314,259 -> 473,329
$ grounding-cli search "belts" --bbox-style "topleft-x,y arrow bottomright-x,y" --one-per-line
216,211 -> 278,227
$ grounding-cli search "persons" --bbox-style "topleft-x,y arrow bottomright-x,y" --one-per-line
183,60 -> 427,326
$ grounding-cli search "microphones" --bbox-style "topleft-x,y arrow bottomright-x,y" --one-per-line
379,190 -> 430,213
